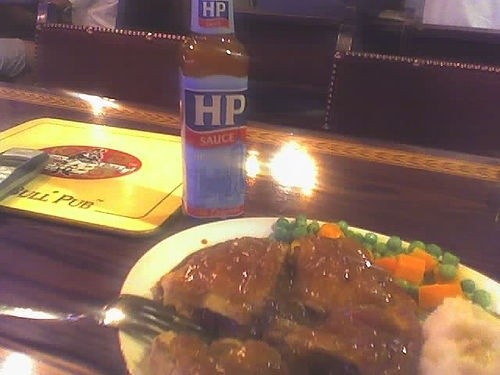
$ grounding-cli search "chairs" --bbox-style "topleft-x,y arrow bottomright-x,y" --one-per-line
330,19 -> 499,157
34,1 -> 185,114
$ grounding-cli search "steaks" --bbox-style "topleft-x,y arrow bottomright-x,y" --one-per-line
150,235 -> 423,375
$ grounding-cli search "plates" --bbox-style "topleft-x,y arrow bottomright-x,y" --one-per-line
115,218 -> 499,375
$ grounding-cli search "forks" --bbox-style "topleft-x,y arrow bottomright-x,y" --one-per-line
0,292 -> 206,337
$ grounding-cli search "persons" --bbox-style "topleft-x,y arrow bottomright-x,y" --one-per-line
0,0 -> 500,85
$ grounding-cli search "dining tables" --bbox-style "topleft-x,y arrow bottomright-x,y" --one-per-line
1,78 -> 500,373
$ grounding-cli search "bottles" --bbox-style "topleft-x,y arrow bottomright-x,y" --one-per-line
177,1 -> 249,218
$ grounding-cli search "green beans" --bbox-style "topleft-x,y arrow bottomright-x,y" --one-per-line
271,215 -> 490,308
